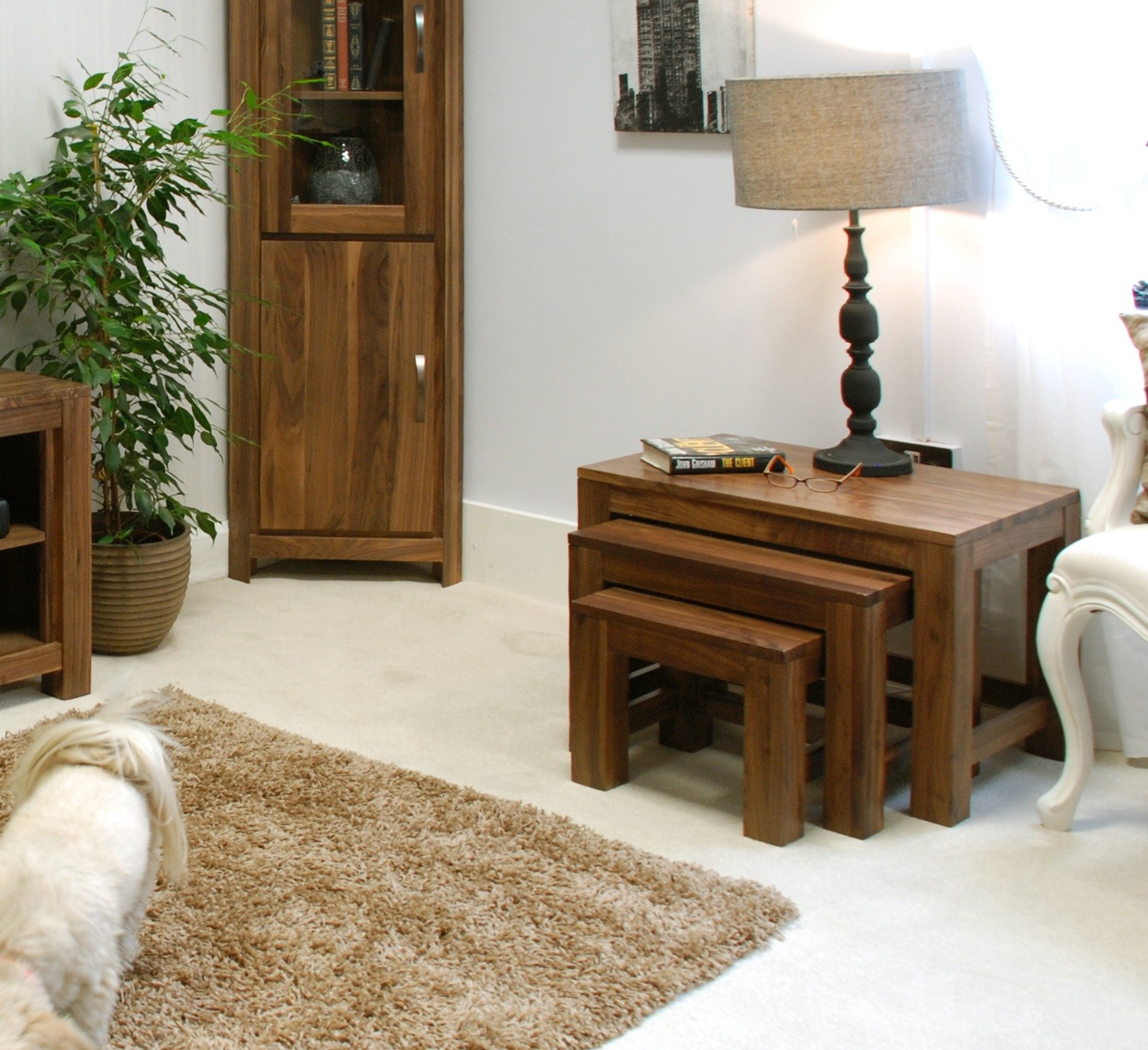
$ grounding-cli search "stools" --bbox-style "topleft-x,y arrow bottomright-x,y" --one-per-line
569,587 -> 822,848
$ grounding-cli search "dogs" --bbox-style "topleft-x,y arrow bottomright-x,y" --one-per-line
0,690 -> 192,1050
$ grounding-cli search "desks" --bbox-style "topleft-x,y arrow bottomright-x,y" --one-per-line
567,430 -> 1080,840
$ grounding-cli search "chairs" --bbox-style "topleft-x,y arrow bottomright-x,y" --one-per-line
1034,281 -> 1148,830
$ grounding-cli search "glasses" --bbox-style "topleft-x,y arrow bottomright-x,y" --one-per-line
764,454 -> 862,493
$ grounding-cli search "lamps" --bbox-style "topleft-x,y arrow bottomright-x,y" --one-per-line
971,475 -> 991,485
728,67 -> 968,481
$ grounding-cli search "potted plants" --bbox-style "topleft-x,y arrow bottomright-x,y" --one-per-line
0,0 -> 336,659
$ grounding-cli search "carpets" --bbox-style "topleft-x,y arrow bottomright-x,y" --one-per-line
0,684 -> 798,1049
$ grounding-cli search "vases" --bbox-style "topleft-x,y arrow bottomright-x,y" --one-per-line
309,137 -> 385,205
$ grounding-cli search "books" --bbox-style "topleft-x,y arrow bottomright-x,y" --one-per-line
640,433 -> 786,475
322,0 -> 365,91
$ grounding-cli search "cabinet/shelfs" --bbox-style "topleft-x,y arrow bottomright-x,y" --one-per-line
0,371 -> 93,702
228,0 -> 464,588
